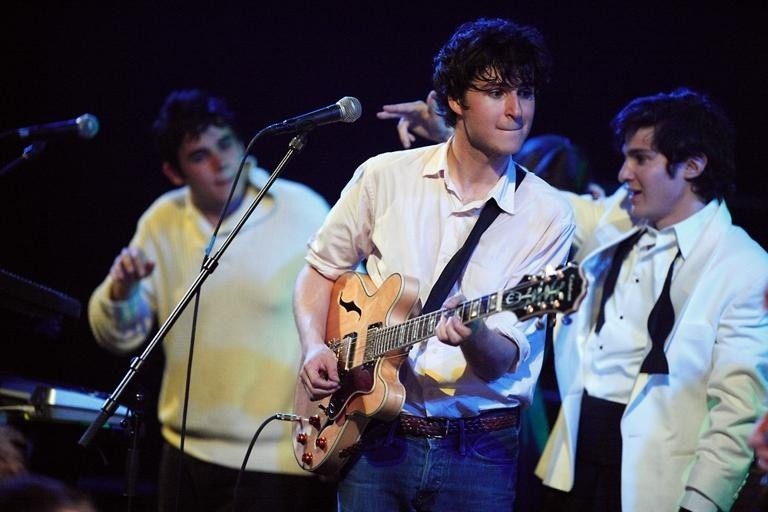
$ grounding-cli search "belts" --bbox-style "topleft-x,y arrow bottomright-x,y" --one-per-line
386,405 -> 529,436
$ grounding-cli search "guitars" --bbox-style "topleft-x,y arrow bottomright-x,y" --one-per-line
291,262 -> 588,476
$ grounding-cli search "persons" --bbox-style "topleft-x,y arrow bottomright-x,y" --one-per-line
375,88 -> 767,510
86,89 -> 364,511
0,475 -> 77,512
1,425 -> 30,482
514,133 -> 607,202
748,281 -> 768,473
293,17 -> 573,512
67,493 -> 97,511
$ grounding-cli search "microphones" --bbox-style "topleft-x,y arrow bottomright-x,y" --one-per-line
269,96 -> 362,135
18,114 -> 99,139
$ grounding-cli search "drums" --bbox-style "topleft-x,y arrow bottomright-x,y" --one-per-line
0,378 -> 132,480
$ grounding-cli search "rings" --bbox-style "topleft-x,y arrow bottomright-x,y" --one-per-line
303,376 -> 309,383
